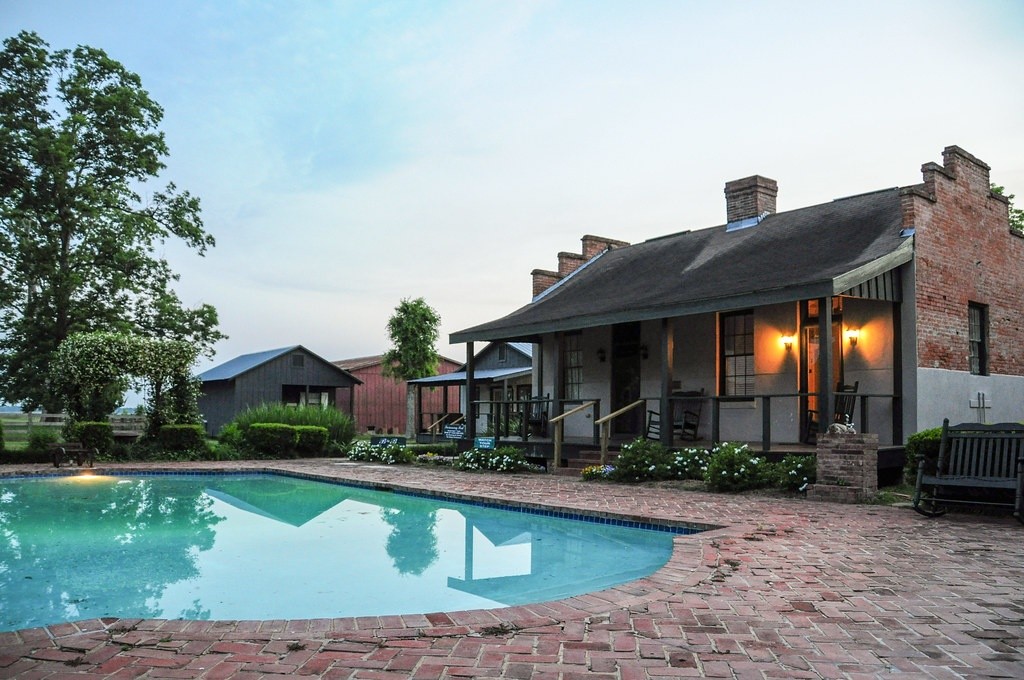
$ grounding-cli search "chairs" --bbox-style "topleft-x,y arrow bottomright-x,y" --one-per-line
642,387 -> 704,442
800,380 -> 859,445
518,393 -> 550,438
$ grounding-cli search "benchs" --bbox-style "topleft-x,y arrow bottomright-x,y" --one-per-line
45,443 -> 100,469
911,418 -> 1024,524
437,413 -> 467,433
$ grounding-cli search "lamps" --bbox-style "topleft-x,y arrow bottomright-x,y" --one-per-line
845,325 -> 859,349
596,347 -> 606,362
783,331 -> 793,355
639,342 -> 649,359
